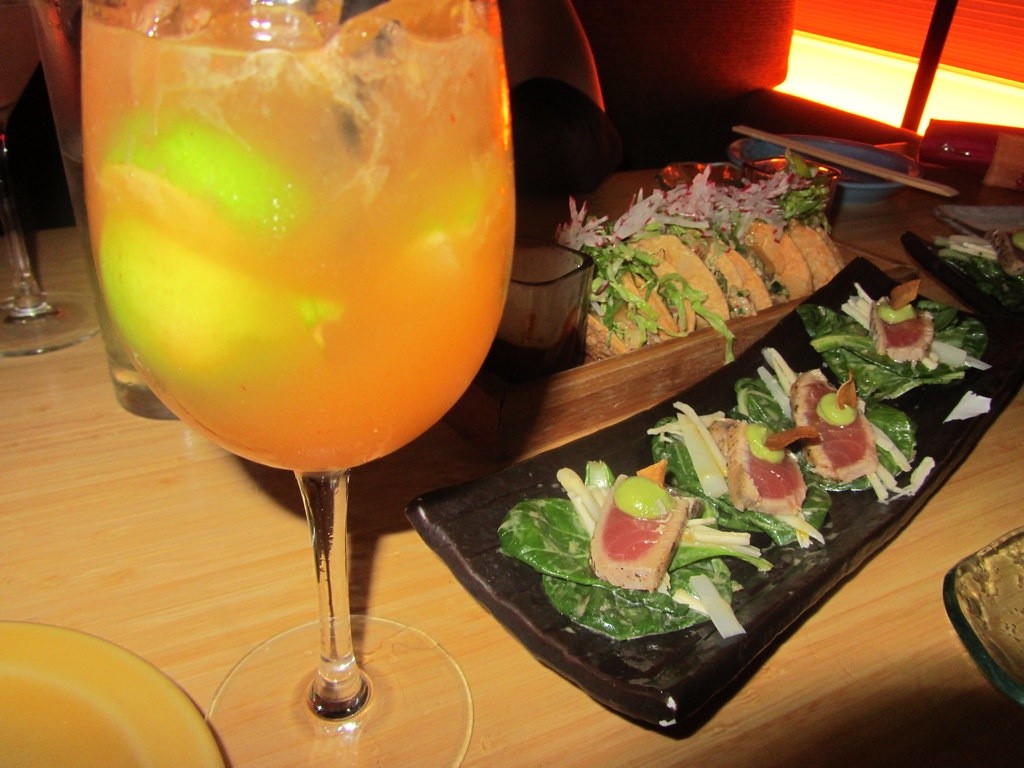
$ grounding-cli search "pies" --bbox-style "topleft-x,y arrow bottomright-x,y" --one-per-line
559,176 -> 843,356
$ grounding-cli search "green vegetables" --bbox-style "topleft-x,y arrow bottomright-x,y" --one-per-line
498,228 -> 1024,644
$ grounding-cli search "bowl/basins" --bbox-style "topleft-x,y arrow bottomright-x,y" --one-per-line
727,136 -> 911,216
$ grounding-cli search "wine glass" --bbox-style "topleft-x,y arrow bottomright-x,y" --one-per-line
0,0 -> 101,355
77,0 -> 520,768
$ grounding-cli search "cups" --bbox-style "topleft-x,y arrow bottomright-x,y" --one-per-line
28,0 -> 184,419
488,235 -> 596,385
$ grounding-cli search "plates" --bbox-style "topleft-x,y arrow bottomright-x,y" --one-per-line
936,206 -> 1024,258
903,231 -> 1024,333
942,526 -> 1024,707
402,256 -> 1024,729
0,622 -> 236,768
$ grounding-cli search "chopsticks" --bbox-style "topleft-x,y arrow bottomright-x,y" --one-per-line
730,123 -> 958,198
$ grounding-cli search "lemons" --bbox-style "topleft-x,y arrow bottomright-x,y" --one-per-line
96,113 -> 308,263
96,216 -> 339,399
